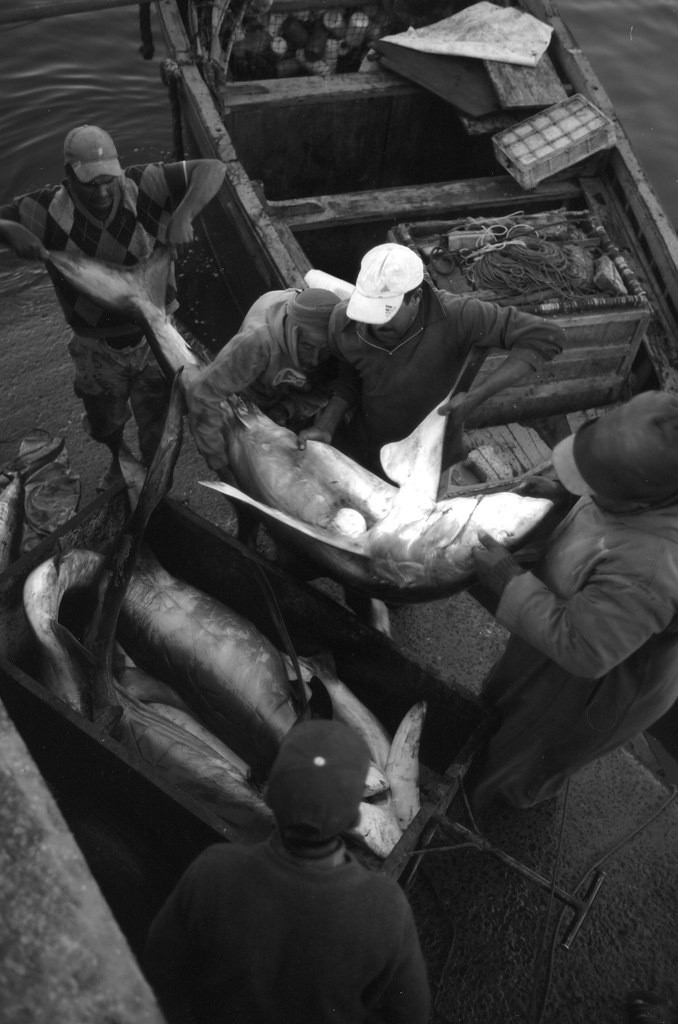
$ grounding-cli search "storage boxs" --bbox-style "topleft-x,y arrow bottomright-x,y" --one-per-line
491,94 -> 617,191
0,477 -> 505,890
388,209 -> 655,427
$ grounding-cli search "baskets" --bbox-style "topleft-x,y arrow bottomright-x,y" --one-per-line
492,93 -> 616,190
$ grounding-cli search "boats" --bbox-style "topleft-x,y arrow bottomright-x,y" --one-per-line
152,0 -> 678,493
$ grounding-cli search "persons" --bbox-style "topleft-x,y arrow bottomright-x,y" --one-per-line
139,718 -> 432,1023
183,244 -> 566,491
1,126 -> 225,486
448,389 -> 678,824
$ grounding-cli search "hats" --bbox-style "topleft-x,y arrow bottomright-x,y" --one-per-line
287,288 -> 342,329
551,389 -> 678,505
346,243 -> 426,326
63,124 -> 122,184
265,718 -> 372,841
